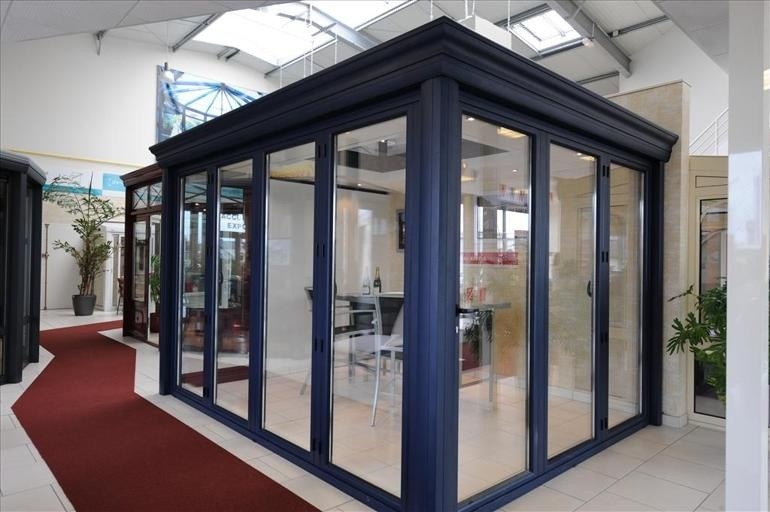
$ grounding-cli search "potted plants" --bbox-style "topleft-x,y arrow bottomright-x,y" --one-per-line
42,171 -> 125,317
147,251 -> 164,334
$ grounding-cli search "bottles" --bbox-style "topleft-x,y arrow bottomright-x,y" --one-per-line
373,265 -> 382,293
362,266 -> 370,294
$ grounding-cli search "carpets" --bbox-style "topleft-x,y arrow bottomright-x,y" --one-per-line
179,364 -> 254,386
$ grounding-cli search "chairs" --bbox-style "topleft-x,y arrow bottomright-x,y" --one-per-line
369,292 -> 405,430
115,275 -> 125,316
297,288 -> 379,402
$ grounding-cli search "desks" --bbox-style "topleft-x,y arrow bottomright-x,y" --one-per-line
345,292 -> 513,405
